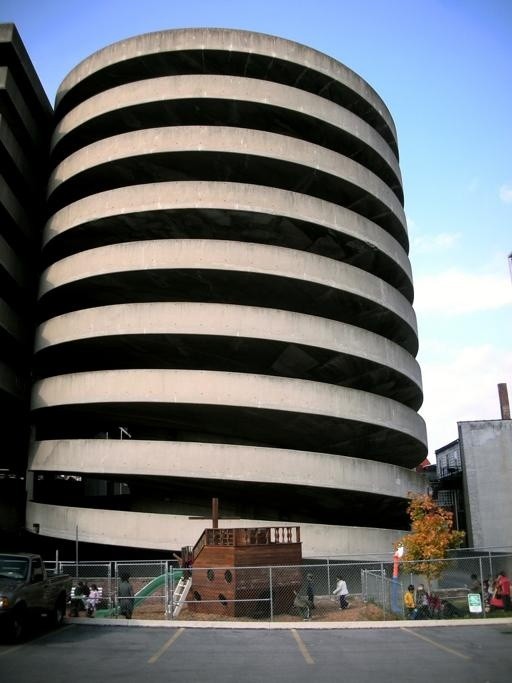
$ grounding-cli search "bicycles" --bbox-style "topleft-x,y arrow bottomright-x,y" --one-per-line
288,588 -> 315,619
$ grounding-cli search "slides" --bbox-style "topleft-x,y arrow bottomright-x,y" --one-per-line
95,571 -> 183,618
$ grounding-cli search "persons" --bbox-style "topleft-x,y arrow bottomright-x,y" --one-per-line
117,571 -> 133,619
464,572 -> 512,613
404,584 -> 429,620
68,579 -> 99,617
333,575 -> 352,609
306,572 -> 315,610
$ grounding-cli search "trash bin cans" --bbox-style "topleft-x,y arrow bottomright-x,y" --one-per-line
68,599 -> 80,617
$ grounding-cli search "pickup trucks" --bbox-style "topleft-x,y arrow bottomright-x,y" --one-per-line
0,552 -> 72,645
